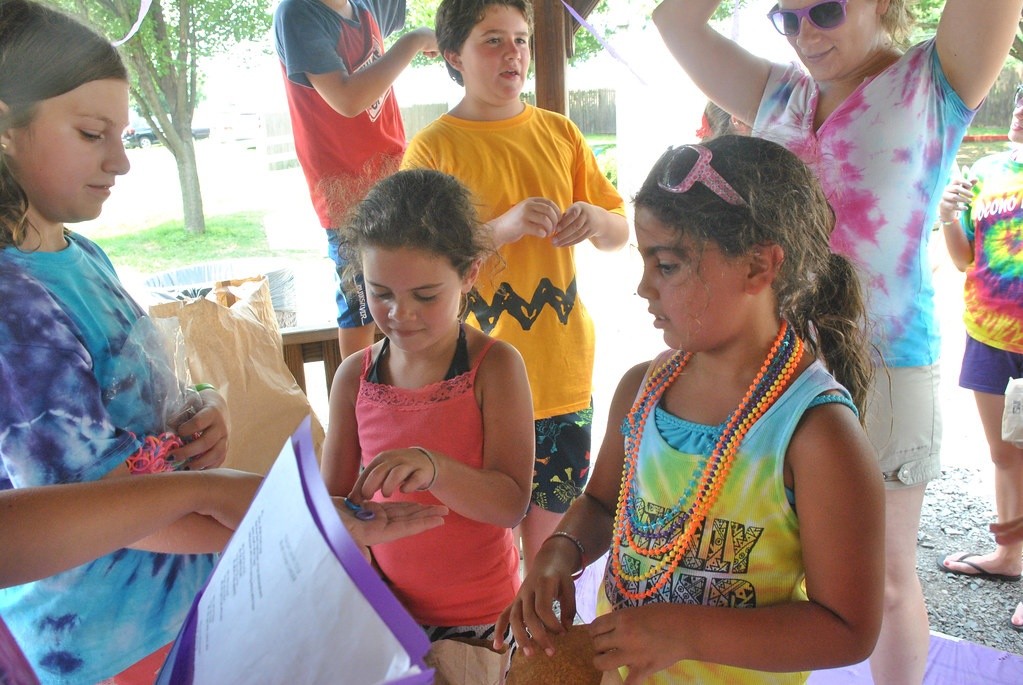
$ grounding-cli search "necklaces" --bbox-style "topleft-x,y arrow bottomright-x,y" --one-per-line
612,317 -> 804,600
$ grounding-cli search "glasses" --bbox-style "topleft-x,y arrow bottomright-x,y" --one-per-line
767,0 -> 848,36
648,142 -> 749,208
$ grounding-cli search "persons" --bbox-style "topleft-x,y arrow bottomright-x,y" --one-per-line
319,170 -> 536,684
399,0 -> 629,624
1,0 -> 448,685
650,0 -> 1022,685
274,0 -> 441,363
492,135 -> 887,684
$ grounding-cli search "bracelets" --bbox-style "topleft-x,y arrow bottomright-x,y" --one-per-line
932,211 -> 959,232
408,446 -> 438,492
542,532 -> 587,581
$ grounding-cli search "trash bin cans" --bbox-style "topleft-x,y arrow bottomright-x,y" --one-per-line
136,256 -> 300,331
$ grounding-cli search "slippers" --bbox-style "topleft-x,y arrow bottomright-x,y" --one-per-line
936,554 -> 1022,582
1008,594 -> 1023,632
